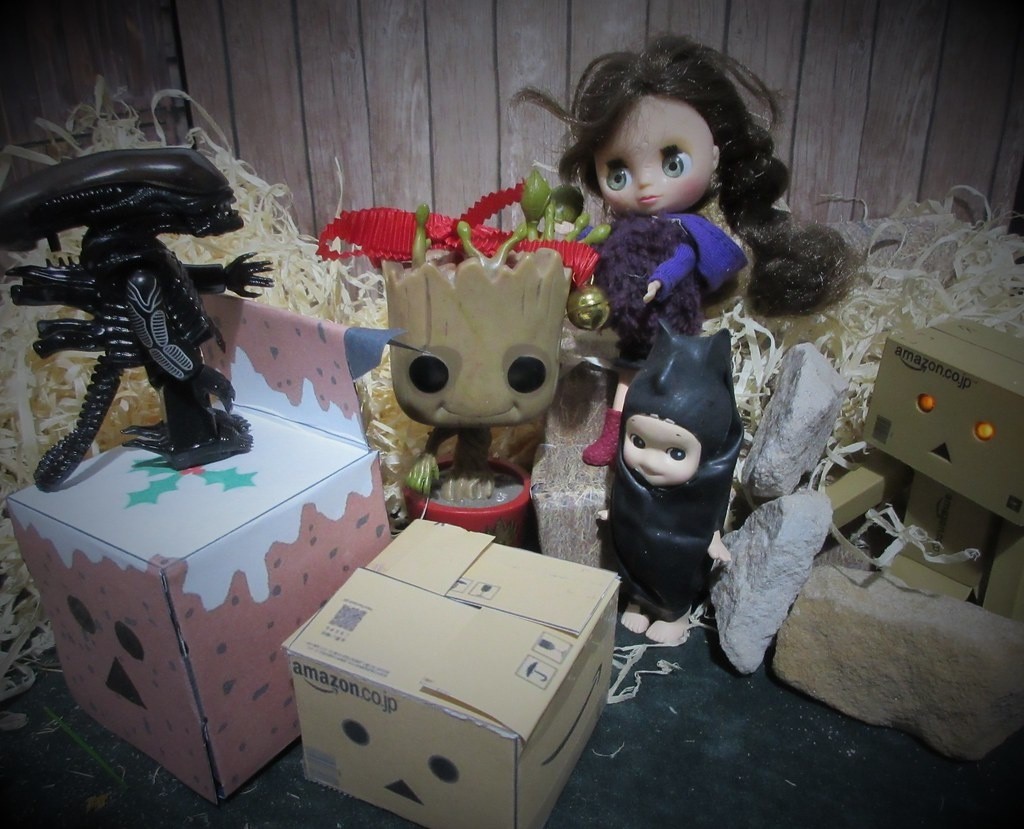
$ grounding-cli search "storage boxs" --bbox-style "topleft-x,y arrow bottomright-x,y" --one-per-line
280,520 -> 620,829
8,292 -> 406,806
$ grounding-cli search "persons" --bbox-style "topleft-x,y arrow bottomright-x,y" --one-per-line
596,316 -> 746,645
507,24 -> 861,467
